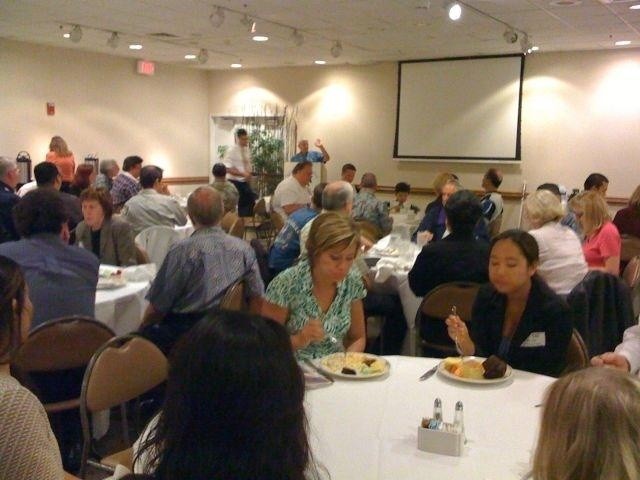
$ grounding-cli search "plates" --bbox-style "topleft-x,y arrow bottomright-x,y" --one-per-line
439,356 -> 514,384
318,352 -> 390,380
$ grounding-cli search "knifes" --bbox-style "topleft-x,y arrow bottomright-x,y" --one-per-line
419,363 -> 439,380
303,357 -> 334,383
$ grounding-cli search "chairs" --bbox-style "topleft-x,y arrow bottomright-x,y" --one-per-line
230,218 -> 245,240
267,205 -> 282,247
134,225 -> 181,262
488,212 -> 505,235
244,197 -> 266,239
76,334 -> 173,480
559,328 -> 591,378
414,281 -> 483,358
14,315 -> 129,451
219,276 -> 250,311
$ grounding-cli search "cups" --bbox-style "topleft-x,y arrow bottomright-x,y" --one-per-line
416,231 -> 428,253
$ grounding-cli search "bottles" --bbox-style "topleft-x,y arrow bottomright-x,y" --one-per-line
433,398 -> 443,423
454,401 -> 466,444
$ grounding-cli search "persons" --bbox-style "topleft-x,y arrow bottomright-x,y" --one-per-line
407,190 -> 491,361
351,173 -> 393,237
537,185 -> 577,237
68,163 -> 94,198
445,229 -> 573,378
74,185 -> 138,270
206,163 -> 238,229
297,182 -> 374,266
385,182 -> 420,214
290,137 -> 329,163
584,173 -> 608,198
589,318 -> 640,381
340,163 -> 360,197
119,167 -> 188,265
20,162 -> 84,245
266,182 -> 329,284
411,181 -> 463,247
534,369 -> 640,480
269,162 -> 314,231
0,188 -> 100,420
47,135 -> 76,195
0,157 -> 25,244
424,172 -> 458,215
611,185 -> 640,278
90,159 -> 117,195
222,128 -> 256,219
119,308 -> 331,480
523,190 -> 588,305
17,178 -> 38,199
569,190 -> 620,278
480,169 -> 505,239
0,252 -> 83,480
110,157 -> 145,213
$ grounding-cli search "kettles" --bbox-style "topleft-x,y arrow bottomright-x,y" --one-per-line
16,150 -> 33,191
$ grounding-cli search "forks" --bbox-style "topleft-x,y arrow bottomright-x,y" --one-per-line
451,305 -> 464,355
324,329 -> 338,345
